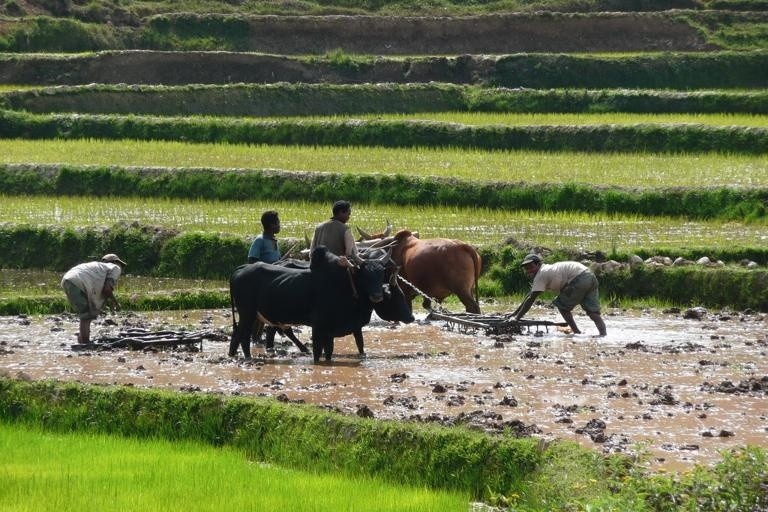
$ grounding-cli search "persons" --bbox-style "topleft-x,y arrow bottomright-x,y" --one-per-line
310,200 -> 363,355
248,210 -> 281,348
507,253 -> 606,335
60,253 -> 128,344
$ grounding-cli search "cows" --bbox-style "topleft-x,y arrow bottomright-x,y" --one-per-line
229,218 -> 483,364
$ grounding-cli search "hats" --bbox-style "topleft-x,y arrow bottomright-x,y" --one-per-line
520,254 -> 539,267
102,254 -> 127,266
